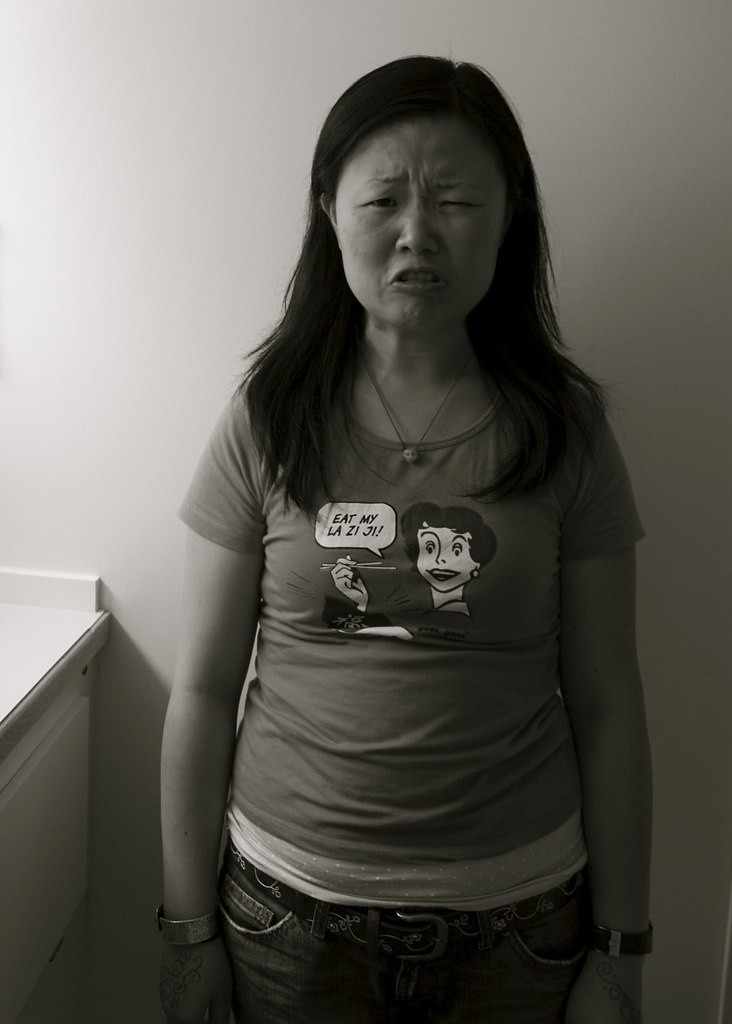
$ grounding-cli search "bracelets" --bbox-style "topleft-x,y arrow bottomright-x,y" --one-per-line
590,921 -> 653,958
155,904 -> 215,945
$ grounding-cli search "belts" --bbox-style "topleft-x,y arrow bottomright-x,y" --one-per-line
226,837 -> 591,961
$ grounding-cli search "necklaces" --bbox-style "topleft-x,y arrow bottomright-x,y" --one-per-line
365,358 -> 463,464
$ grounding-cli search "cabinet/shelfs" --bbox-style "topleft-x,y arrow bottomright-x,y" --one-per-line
0,568 -> 111,1024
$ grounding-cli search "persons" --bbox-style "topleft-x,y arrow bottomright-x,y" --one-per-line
156,55 -> 652,1024
